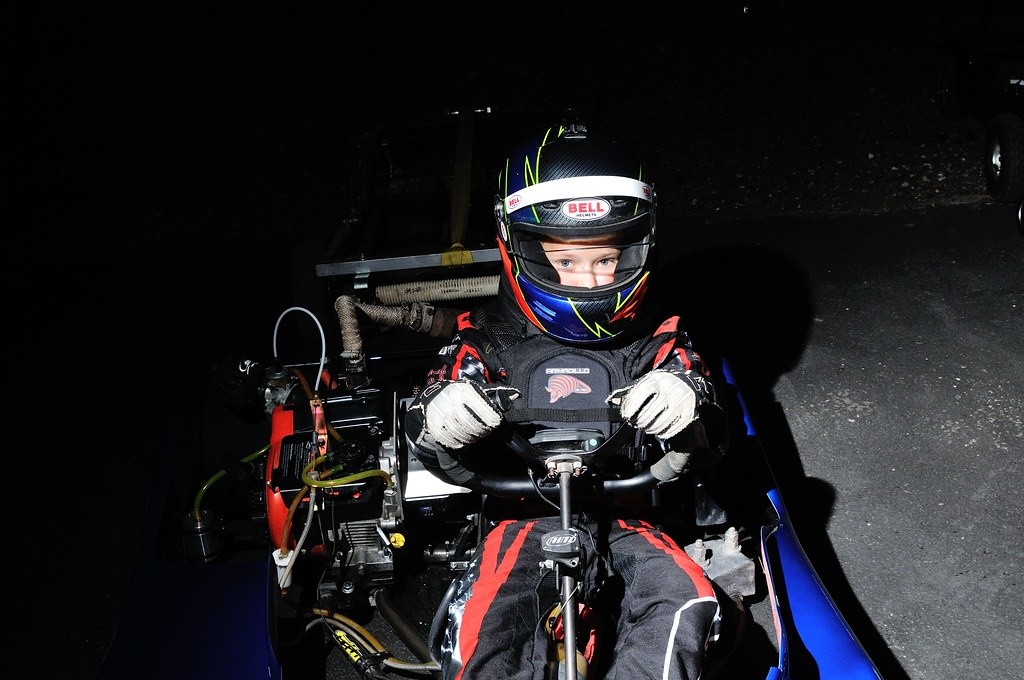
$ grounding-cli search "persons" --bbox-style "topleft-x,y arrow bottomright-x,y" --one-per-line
404,120 -> 750,680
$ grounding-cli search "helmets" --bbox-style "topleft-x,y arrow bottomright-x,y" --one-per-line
493,117 -> 658,350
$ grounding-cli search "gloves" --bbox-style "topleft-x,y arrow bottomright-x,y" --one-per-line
607,368 -> 696,442
424,380 -> 524,452
